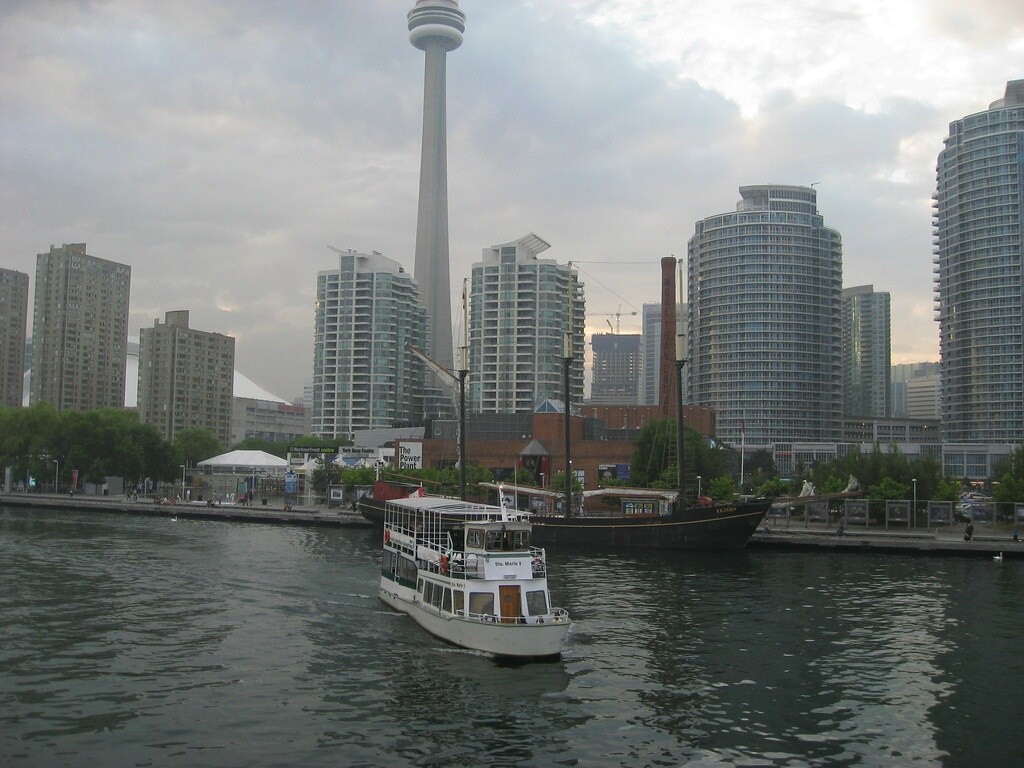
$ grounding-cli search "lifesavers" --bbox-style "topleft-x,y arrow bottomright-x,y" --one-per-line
440,555 -> 447,575
155,507 -> 161,514
384,529 -> 390,545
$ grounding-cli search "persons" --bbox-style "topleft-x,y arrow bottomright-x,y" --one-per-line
126,481 -> 293,511
1012,527 -> 1019,542
837,524 -> 844,537
763,517 -> 772,536
551,613 -> 562,622
963,521 -> 975,542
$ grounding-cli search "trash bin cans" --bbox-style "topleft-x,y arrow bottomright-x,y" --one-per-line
198,495 -> 202,500
262,499 -> 267,505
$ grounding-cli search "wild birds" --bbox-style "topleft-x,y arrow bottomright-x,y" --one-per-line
170,516 -> 177,522
993,552 -> 1002,559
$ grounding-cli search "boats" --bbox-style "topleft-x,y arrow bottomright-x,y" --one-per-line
378,494 -> 572,657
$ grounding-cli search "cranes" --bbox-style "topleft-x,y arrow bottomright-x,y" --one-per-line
585,304 -> 638,335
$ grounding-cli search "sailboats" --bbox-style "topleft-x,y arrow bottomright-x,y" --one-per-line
355,349 -> 775,551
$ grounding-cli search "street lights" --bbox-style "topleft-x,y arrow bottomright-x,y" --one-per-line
912,479 -> 917,527
53,460 -> 58,495
696,476 -> 702,497
180,465 -> 185,500
539,472 -> 545,489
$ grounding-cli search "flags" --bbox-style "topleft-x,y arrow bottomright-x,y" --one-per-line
405,488 -> 423,499
